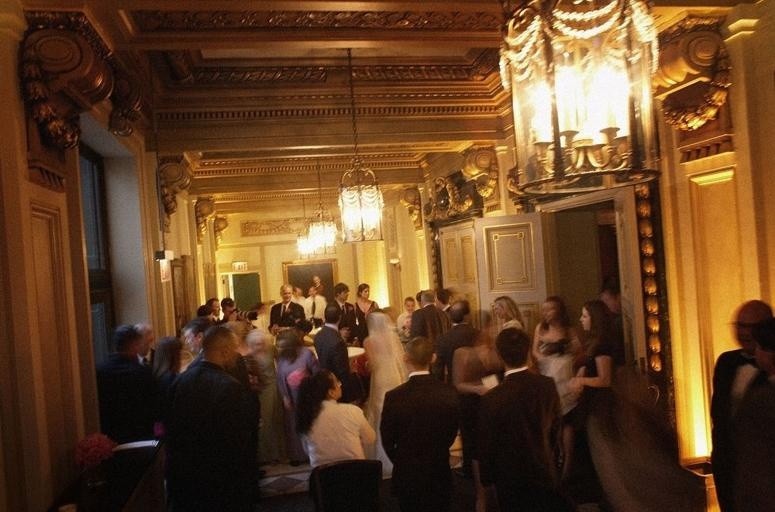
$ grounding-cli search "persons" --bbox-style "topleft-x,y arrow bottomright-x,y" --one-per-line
93,281 -> 773,510
307,276 -> 326,295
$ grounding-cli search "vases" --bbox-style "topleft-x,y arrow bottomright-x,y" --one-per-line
83,465 -> 109,488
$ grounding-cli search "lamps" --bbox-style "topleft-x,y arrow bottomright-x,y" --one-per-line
311,156 -> 338,254
498,0 -> 662,195
339,47 -> 383,243
295,193 -> 319,259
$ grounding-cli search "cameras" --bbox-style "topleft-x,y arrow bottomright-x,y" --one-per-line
233,309 -> 257,321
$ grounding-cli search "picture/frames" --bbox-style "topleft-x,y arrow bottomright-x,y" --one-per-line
282,258 -> 341,313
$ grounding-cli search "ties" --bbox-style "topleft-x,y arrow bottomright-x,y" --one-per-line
143,357 -> 147,366
282,305 -> 286,316
342,305 -> 345,312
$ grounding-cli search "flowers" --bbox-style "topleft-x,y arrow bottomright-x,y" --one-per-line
69,432 -> 118,464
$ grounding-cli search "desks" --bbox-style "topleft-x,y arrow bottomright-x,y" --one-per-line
48,437 -> 167,511
296,344 -> 367,399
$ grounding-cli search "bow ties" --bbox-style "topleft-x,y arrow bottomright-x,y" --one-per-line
737,354 -> 757,366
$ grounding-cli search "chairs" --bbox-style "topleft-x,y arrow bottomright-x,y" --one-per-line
303,457 -> 385,510
